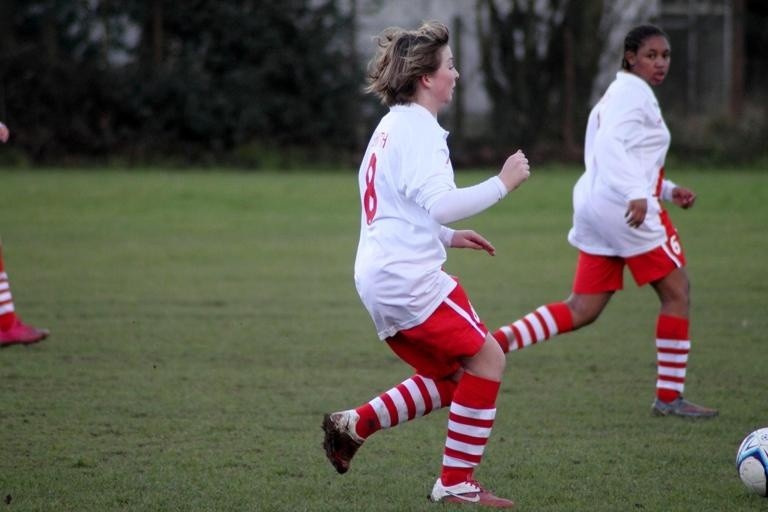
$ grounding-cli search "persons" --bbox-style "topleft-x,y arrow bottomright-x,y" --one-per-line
0,122 -> 51,345
491,23 -> 719,419
320,20 -> 531,507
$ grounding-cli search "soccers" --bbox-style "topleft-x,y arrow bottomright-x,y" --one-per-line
737,428 -> 767,497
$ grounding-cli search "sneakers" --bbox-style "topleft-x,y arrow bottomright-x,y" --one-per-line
0,324 -> 49,347
649,396 -> 718,419
428,477 -> 514,508
322,409 -> 366,475
